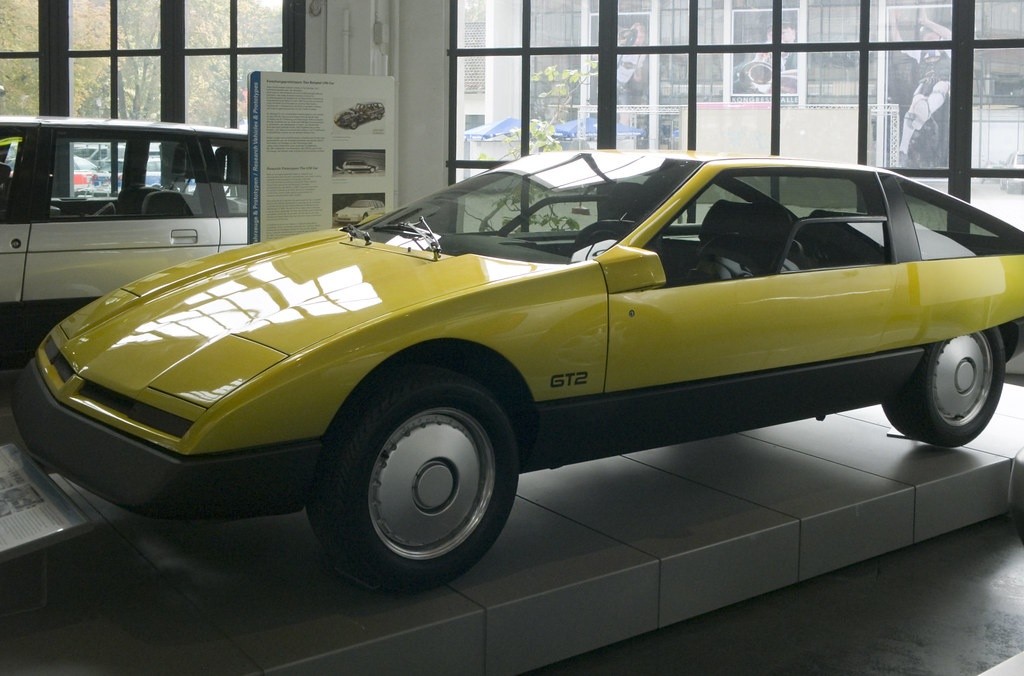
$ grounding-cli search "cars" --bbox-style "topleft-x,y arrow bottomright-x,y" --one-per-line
335,200 -> 384,224
334,100 -> 386,129
343,161 -> 378,173
0,115 -> 250,358
73,154 -> 97,195
999,149 -> 1024,193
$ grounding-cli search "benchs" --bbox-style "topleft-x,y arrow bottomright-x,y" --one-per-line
683,199 -> 891,286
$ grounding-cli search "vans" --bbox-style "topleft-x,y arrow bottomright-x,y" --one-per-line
88,156 -> 161,194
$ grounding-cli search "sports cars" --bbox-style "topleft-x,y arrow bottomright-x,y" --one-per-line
12,148 -> 1024,590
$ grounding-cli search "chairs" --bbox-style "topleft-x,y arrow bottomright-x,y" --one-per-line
117,184 -> 199,216
0,162 -> 13,223
569,181 -> 665,261
